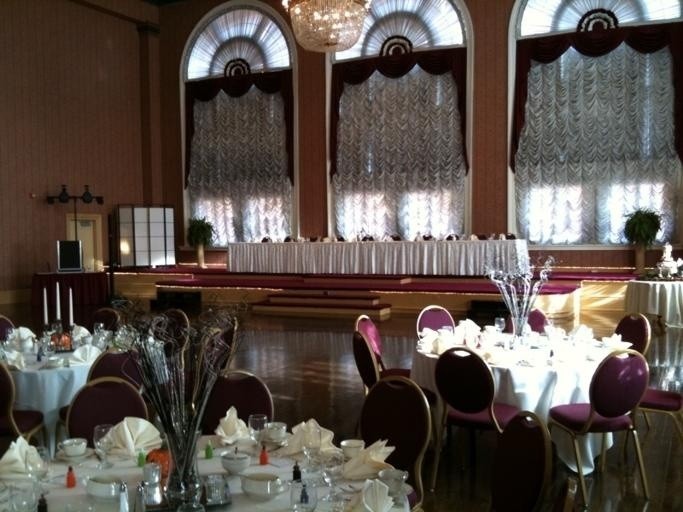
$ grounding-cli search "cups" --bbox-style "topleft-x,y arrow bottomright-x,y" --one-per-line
378,468 -> 409,491
57,438 -> 88,455
341,440 -> 364,459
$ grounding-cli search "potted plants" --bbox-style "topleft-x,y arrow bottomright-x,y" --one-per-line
622,207 -> 663,277
186,212 -> 218,271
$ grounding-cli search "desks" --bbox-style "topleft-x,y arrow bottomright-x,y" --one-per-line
226,238 -> 529,278
628,274 -> 683,376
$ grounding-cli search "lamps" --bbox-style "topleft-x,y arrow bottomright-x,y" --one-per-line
287,0 -> 372,54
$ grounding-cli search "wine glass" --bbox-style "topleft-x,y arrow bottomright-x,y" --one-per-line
92,424 -> 115,469
289,479 -> 319,511
24,447 -> 52,495
300,426 -> 322,473
247,415 -> 269,451
5,322 -> 131,367
420,318 -> 632,369
320,453 -> 345,502
14,478 -> 37,510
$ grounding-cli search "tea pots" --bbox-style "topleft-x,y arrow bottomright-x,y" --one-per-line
132,479 -> 169,512
657,242 -> 683,275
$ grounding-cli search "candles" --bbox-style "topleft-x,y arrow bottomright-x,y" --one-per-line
42,280 -> 74,326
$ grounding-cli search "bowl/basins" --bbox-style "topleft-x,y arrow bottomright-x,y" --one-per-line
221,450 -> 251,475
240,468 -> 283,499
87,475 -> 125,500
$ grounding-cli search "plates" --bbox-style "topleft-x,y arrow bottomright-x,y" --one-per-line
389,483 -> 413,498
56,447 -> 95,460
